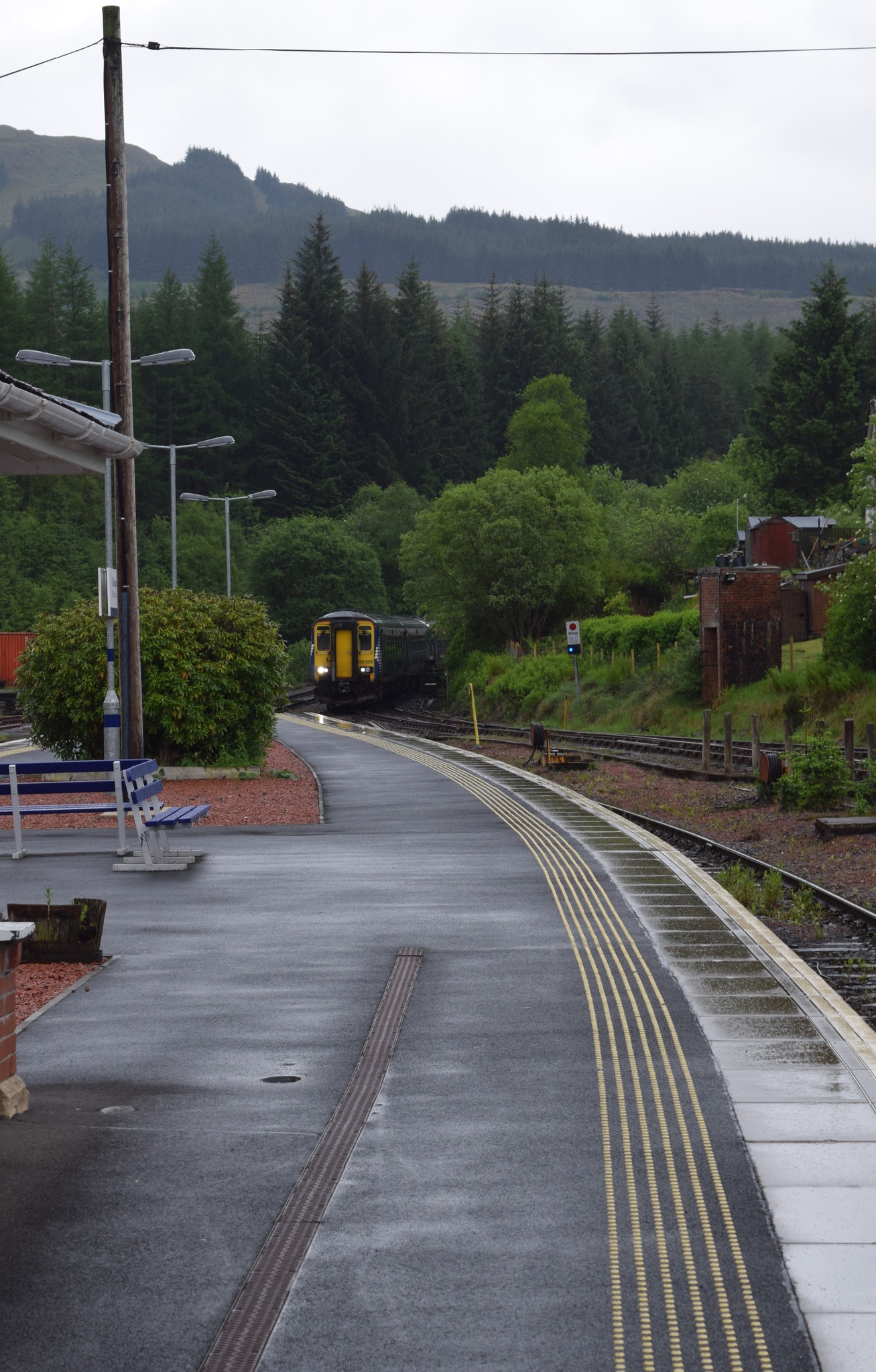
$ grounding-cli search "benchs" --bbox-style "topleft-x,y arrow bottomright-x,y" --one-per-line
0,759 -> 211,871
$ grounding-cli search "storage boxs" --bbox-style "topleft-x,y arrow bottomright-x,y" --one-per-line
0,633 -> 37,685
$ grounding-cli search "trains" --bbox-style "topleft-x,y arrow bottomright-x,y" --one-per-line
311,609 -> 444,718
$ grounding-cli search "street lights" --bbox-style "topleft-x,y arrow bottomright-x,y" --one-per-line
178,488 -> 278,596
17,344 -> 194,759
136,435 -> 236,588
